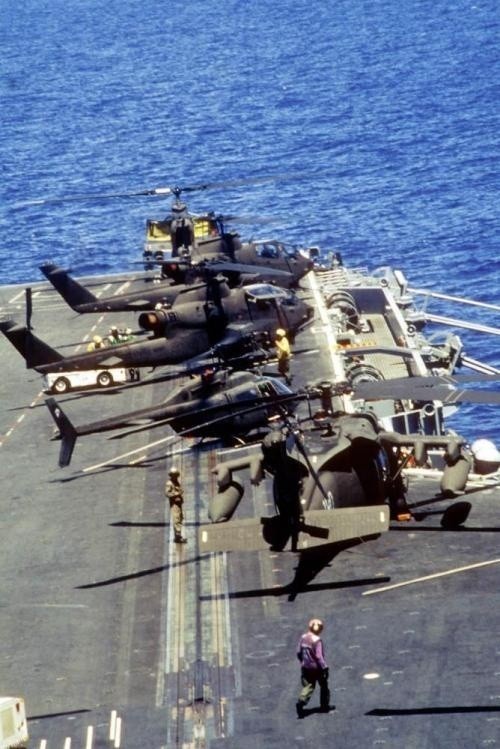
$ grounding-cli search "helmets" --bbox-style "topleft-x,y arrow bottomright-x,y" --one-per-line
310,620 -> 323,633
168,467 -> 180,477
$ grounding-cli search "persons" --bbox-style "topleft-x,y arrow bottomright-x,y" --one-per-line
107,326 -> 125,347
295,619 -> 337,719
87,336 -> 105,353
274,326 -> 293,386
121,328 -> 141,383
163,465 -> 187,545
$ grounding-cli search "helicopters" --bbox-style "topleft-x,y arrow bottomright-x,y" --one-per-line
2,177 -> 499,560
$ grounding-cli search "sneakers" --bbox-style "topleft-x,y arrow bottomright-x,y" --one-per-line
296,703 -> 304,718
173,537 -> 188,543
320,705 -> 335,711
